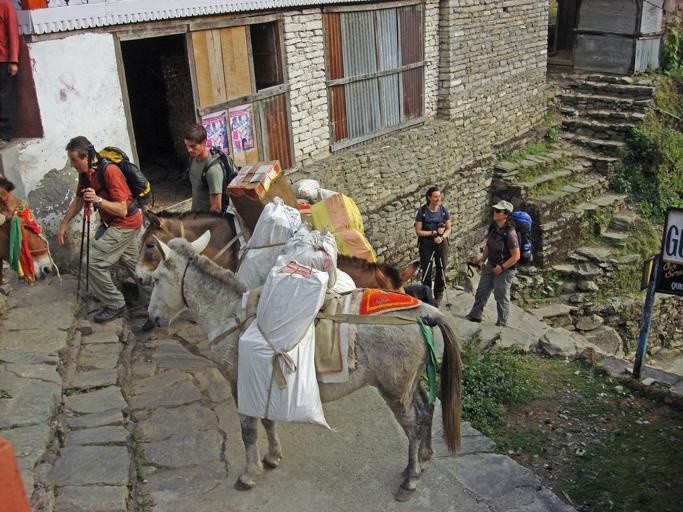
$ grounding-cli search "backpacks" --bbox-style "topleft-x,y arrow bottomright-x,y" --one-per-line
186,146 -> 240,206
79,146 -> 154,221
511,211 -> 534,265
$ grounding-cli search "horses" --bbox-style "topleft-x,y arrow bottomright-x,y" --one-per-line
133,209 -> 464,502
0,173 -> 54,286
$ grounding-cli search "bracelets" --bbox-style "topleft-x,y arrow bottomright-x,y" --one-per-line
498,263 -> 505,272
95,196 -> 103,207
440,235 -> 444,240
431,229 -> 434,236
436,229 -> 439,235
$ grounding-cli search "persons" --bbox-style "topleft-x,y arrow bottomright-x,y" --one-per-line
181,123 -> 224,214
465,199 -> 521,328
0,0 -> 21,147
60,135 -> 145,325
414,185 -> 453,308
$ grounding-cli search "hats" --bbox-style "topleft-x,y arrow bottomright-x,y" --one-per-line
492,200 -> 514,214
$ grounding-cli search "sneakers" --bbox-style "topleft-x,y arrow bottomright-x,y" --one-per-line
93,304 -> 160,332
465,314 -> 508,326
434,297 -> 443,306
